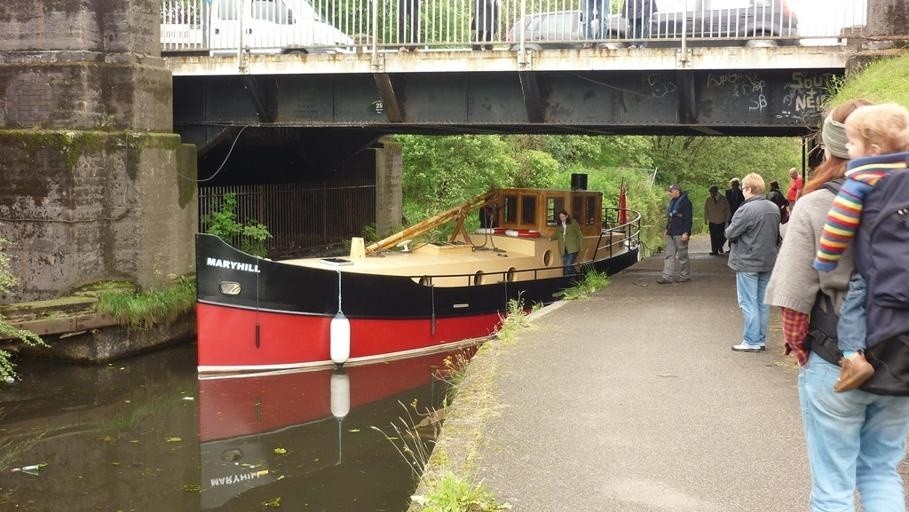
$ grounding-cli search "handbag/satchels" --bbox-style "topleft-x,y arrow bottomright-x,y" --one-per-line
779,206 -> 788,224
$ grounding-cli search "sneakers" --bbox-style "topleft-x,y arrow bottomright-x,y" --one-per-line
675,274 -> 690,282
657,275 -> 673,284
834,352 -> 875,392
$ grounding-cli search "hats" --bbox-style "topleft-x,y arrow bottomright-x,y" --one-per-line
668,183 -> 681,191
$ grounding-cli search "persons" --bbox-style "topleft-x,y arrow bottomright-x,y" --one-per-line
812,102 -> 909,395
621,0 -> 660,49
764,180 -> 789,247
788,166 -> 803,215
722,172 -> 781,351
578,0 -> 613,47
396,0 -> 419,53
656,183 -> 693,284
756,97 -> 909,512
545,208 -> 587,276
470,0 -> 499,51
726,176 -> 746,254
703,185 -> 734,257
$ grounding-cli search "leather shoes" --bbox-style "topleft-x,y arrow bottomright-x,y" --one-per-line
708,246 -> 730,255
732,340 -> 761,353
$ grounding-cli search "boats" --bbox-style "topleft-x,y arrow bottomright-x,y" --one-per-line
190,171 -> 648,381
194,337 -> 496,511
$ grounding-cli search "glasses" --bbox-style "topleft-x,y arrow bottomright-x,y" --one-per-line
819,141 -> 824,150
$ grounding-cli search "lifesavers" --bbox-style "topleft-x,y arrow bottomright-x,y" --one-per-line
505,228 -> 541,239
475,226 -> 507,235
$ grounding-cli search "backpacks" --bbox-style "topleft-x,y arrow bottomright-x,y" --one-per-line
850,167 -> 907,348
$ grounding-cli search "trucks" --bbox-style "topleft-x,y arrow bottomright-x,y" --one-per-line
156,0 -> 357,57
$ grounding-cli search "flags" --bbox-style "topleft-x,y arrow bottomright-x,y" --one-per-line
617,176 -> 628,228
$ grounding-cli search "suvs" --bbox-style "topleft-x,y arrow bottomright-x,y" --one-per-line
573,0 -> 805,48
505,9 -> 612,50
